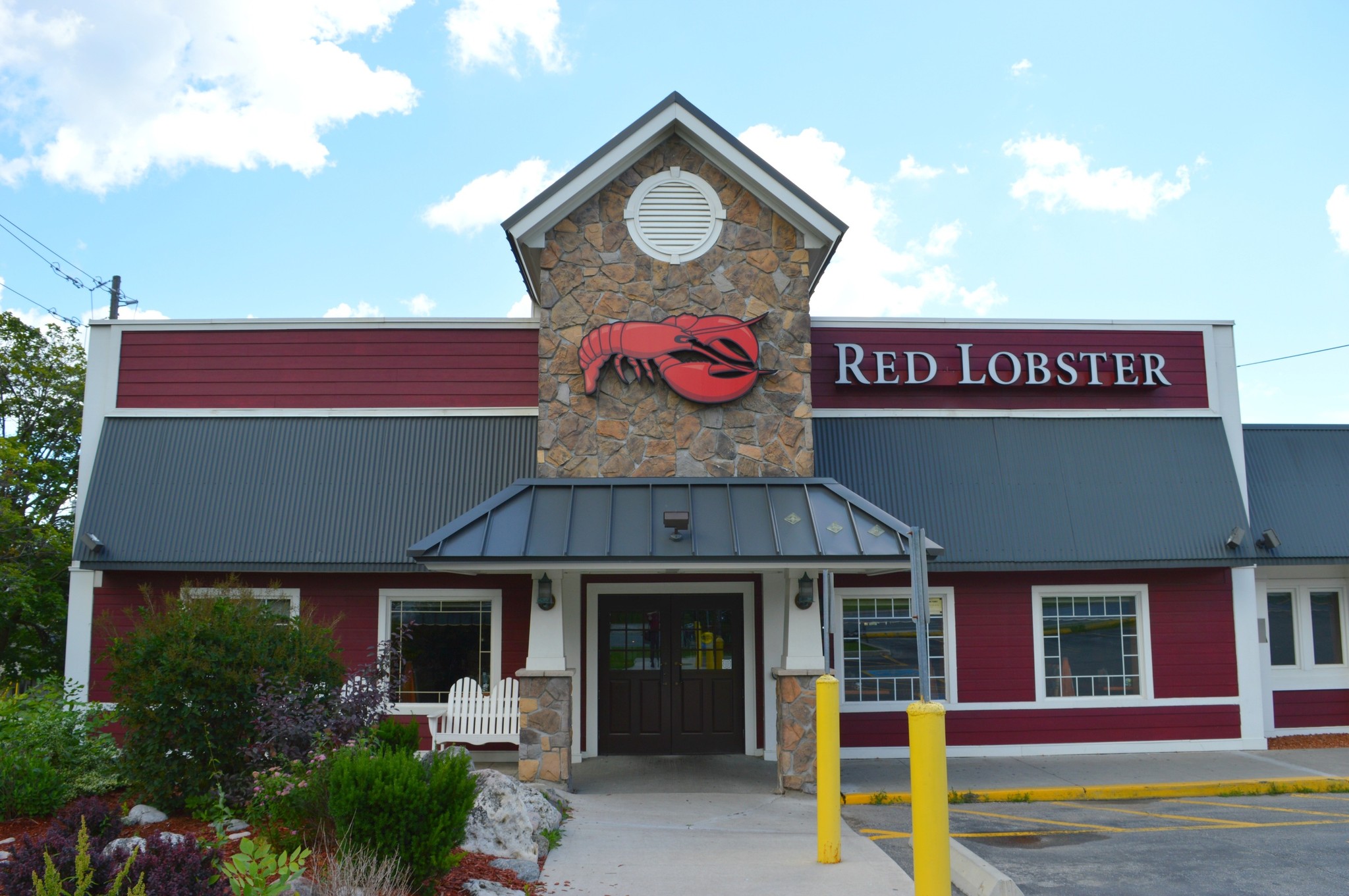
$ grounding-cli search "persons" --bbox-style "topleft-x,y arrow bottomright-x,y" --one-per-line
645,611 -> 660,667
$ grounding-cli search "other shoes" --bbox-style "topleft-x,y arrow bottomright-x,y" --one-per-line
650,665 -> 655,668
657,665 -> 659,667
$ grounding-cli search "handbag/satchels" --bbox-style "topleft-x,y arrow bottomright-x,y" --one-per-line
643,630 -> 651,644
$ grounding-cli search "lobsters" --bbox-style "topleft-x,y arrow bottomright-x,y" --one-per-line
577,311 -> 779,404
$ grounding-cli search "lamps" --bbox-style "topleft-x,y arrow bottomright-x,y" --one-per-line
1226,526 -> 1246,547
664,512 -> 689,541
535,572 -> 555,610
1255,529 -> 1282,551
80,531 -> 104,553
794,571 -> 815,610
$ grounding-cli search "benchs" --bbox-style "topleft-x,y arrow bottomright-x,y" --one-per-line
427,677 -> 520,752
